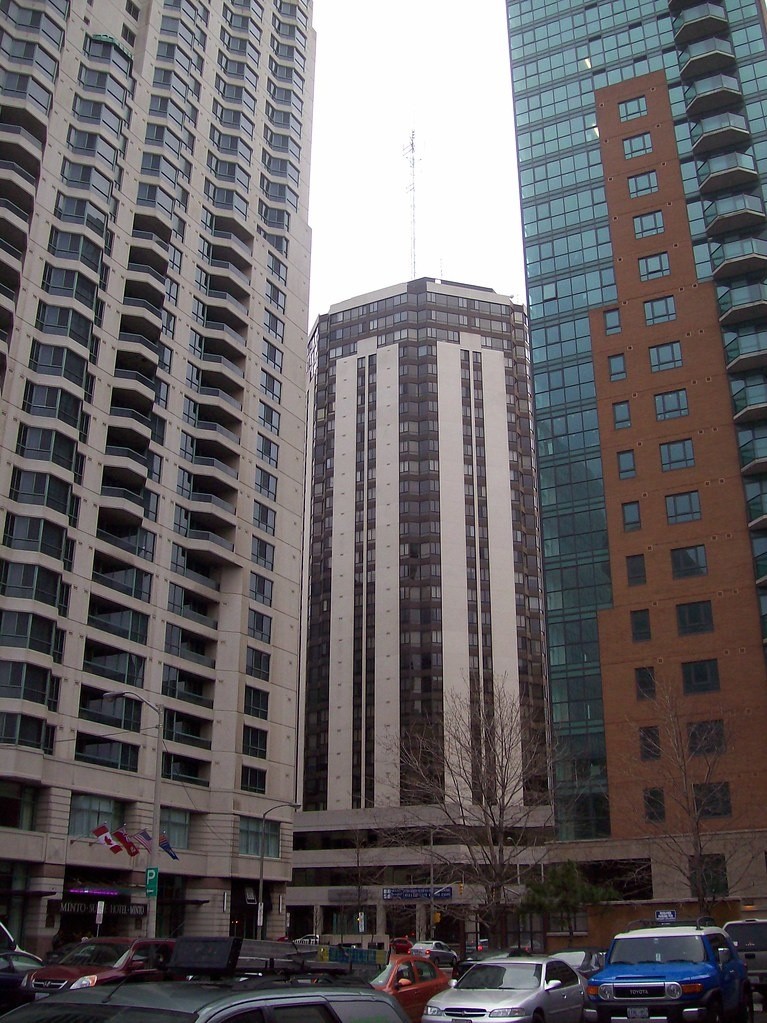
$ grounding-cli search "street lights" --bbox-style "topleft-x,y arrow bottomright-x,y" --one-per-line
507,837 -> 524,948
101,689 -> 164,938
256,803 -> 302,941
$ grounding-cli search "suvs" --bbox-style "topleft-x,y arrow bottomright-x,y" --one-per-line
0,966 -> 408,1023
586,916 -> 753,1022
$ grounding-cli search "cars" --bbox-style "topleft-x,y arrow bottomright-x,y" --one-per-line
407,940 -> 458,967
311,954 -> 452,1023
546,947 -> 608,978
277,936 -> 288,941
389,937 -> 413,954
294,933 -> 319,945
452,948 -> 531,980
18,935 -> 189,1002
420,956 -> 590,1023
0,949 -> 44,1018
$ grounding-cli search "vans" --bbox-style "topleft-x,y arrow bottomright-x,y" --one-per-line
723,918 -> 767,995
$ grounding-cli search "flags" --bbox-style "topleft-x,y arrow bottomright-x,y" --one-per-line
131,828 -> 161,854
91,822 -> 123,854
159,832 -> 180,861
112,824 -> 140,858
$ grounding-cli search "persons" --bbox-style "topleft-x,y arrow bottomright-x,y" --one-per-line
52,928 -> 94,952
